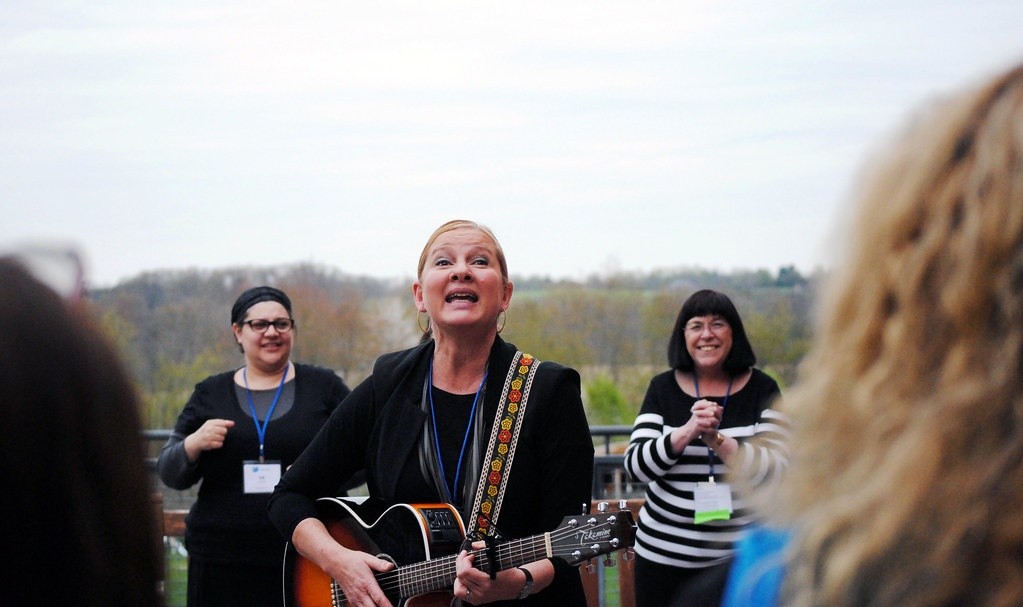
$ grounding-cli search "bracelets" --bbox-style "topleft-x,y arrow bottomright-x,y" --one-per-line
708,432 -> 724,452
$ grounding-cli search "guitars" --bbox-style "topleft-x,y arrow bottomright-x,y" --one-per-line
283,496 -> 639,606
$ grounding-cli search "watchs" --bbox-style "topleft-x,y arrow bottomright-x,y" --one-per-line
671,63 -> 1023,606
515,566 -> 534,601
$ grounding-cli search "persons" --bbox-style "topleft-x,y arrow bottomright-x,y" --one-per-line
623,289 -> 803,606
264,218 -> 595,606
157,285 -> 354,606
0,242 -> 165,607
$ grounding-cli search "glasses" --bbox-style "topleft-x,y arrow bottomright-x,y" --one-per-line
682,321 -> 730,333
240,316 -> 294,333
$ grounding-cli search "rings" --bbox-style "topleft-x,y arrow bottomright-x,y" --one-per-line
463,587 -> 471,601
